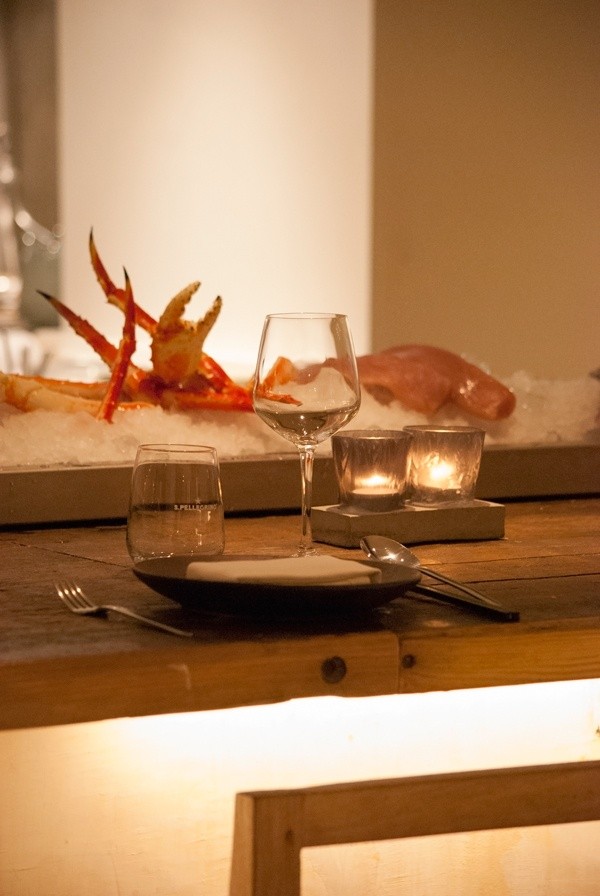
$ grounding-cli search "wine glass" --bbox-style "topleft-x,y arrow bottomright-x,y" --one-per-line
252,312 -> 361,557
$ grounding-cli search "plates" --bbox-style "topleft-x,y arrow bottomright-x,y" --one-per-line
132,555 -> 422,617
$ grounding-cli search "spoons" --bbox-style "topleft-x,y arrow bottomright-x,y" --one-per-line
360,535 -> 505,609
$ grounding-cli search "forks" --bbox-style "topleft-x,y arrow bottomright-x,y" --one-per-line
51,578 -> 194,637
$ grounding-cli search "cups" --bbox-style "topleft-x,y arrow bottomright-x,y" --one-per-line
330,429 -> 411,512
402,424 -> 486,507
126,444 -> 225,562
